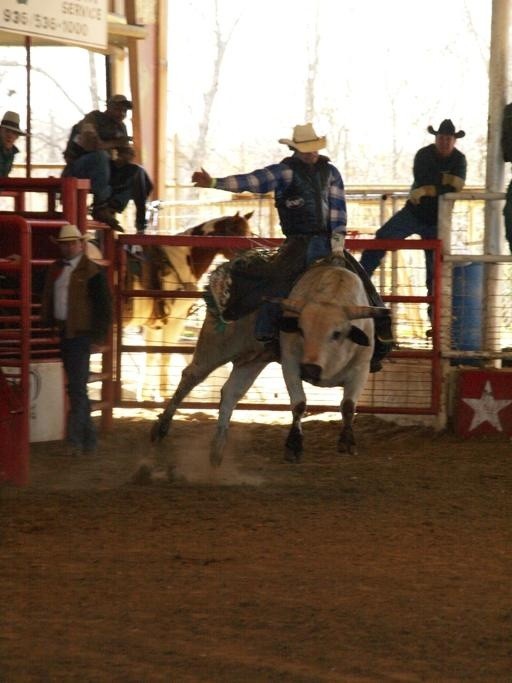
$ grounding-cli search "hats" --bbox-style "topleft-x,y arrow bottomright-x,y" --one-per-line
49,225 -> 90,244
279,124 -> 326,153
428,119 -> 465,139
107,95 -> 132,110
0,111 -> 26,137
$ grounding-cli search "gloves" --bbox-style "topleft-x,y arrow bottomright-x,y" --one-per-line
409,187 -> 425,205
452,175 -> 464,191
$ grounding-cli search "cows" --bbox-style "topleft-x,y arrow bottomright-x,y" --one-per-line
149,261 -> 393,470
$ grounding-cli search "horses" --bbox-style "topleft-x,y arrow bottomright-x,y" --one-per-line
86,209 -> 256,412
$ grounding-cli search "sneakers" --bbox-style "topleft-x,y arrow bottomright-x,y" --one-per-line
91,209 -> 124,233
47,441 -> 96,456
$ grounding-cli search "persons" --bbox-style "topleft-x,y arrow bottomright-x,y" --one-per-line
40,226 -> 114,458
191,122 -> 383,374
359,119 -> 467,336
501,101 -> 512,258
0,110 -> 30,177
60,93 -> 153,234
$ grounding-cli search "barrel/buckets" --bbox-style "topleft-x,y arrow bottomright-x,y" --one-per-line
450,248 -> 485,366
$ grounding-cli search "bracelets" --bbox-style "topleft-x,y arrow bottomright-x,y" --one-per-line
210,178 -> 216,188
